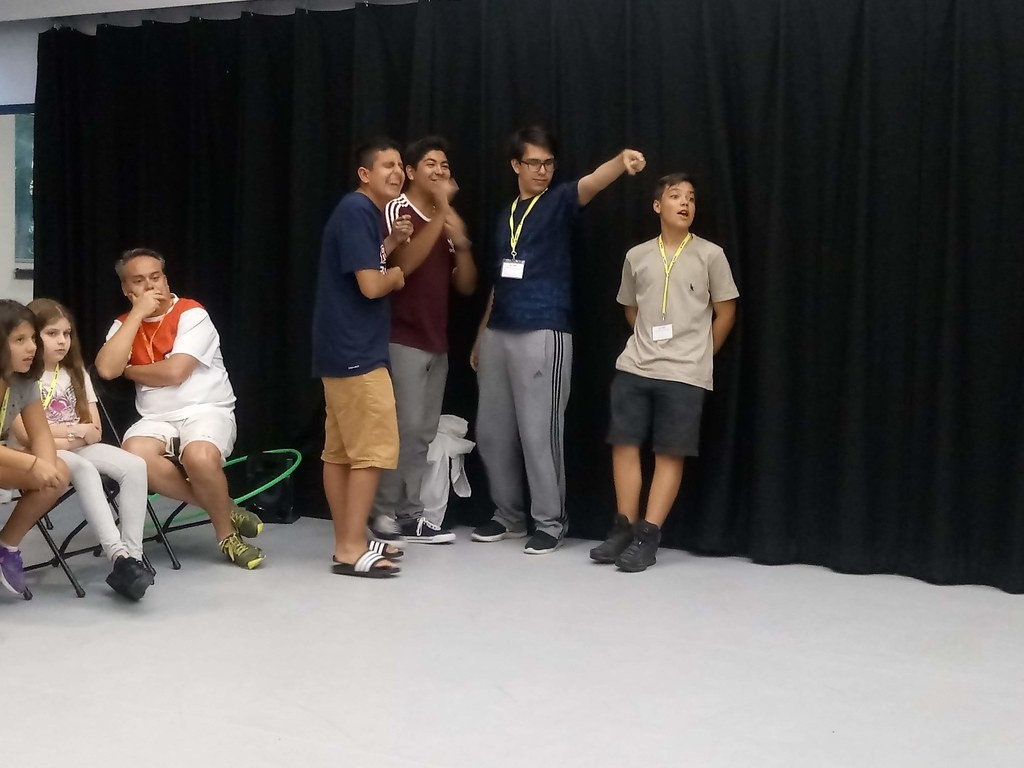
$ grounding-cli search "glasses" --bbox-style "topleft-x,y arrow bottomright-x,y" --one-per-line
518,158 -> 556,172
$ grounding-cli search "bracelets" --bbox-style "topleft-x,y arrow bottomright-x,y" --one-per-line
28,456 -> 38,472
125,364 -> 132,367
65,433 -> 75,450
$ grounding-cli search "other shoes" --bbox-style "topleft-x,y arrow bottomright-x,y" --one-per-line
106,557 -> 145,595
115,555 -> 154,599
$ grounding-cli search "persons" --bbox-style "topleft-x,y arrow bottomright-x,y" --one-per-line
307,139 -> 414,578
94,247 -> 266,569
0,298 -> 71,594
367,137 -> 474,547
591,173 -> 739,571
7,297 -> 154,602
469,124 -> 648,554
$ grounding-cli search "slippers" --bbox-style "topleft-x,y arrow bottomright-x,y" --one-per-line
332,550 -> 401,577
331,539 -> 403,562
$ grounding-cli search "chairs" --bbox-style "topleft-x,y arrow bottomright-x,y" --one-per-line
0,383 -> 181,601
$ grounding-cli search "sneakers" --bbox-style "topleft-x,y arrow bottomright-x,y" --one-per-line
615,519 -> 662,572
471,520 -> 527,542
218,532 -> 266,569
0,544 -> 25,595
228,497 -> 263,538
366,515 -> 407,547
398,517 -> 456,544
524,529 -> 565,554
590,512 -> 640,563
